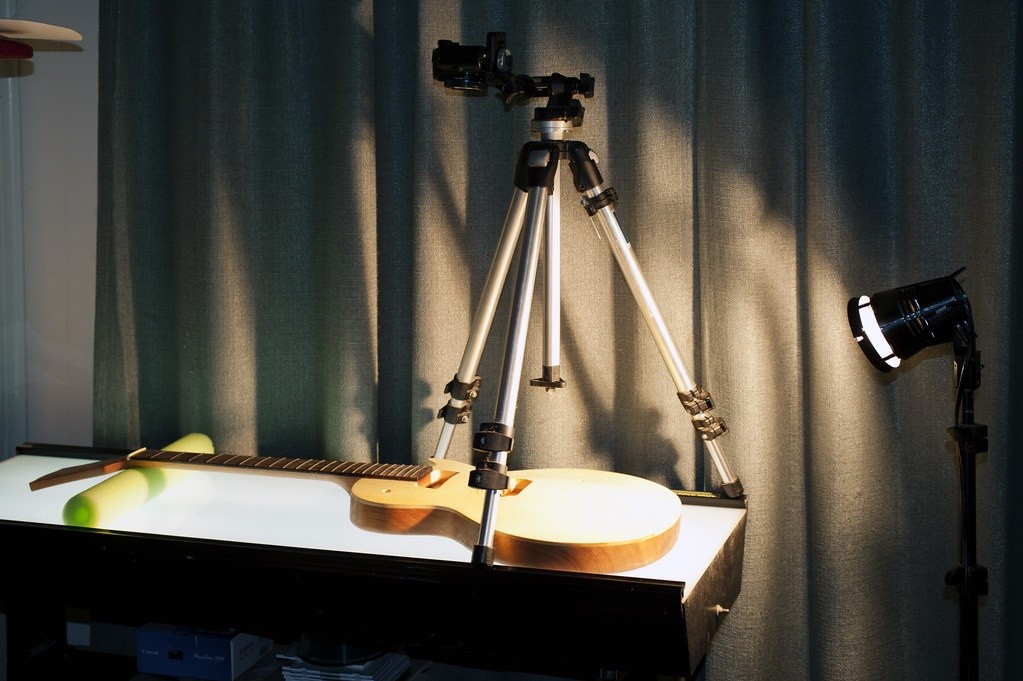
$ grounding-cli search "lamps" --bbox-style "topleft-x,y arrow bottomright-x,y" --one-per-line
847,265 -> 990,681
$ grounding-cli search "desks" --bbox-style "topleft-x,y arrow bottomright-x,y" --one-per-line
0,454 -> 749,681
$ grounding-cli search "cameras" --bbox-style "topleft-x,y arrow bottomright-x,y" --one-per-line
431,29 -> 522,100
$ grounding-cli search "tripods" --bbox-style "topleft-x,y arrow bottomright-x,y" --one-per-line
427,72 -> 744,566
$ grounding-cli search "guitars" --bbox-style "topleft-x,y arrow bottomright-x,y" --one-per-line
14,441 -> 682,574
